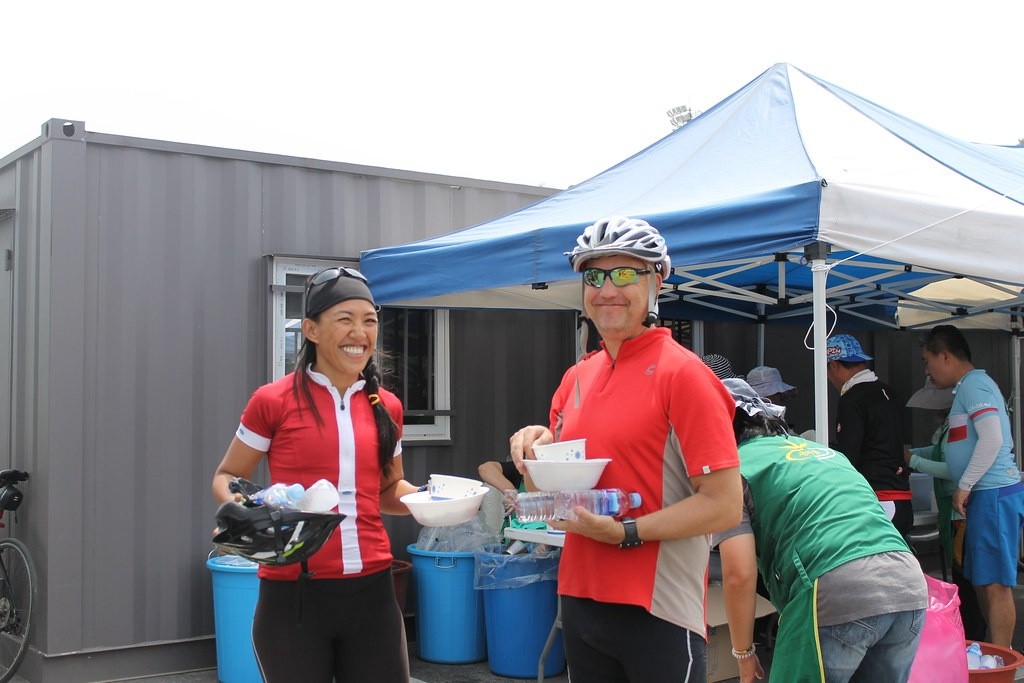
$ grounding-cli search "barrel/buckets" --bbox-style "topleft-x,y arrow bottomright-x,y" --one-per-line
909,474 -> 932,511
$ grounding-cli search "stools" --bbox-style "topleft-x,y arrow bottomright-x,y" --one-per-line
908,525 -> 948,583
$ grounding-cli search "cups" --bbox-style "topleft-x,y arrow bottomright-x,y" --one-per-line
300,479 -> 339,515
507,541 -> 525,555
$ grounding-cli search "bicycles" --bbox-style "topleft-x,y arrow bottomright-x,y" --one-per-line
0,470 -> 38,683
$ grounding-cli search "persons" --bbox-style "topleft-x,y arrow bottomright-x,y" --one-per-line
510,215 -> 743,683
712,378 -> 929,683
212,267 -> 429,683
921,325 -> 1024,649
699,335 -> 987,642
478,461 -> 557,552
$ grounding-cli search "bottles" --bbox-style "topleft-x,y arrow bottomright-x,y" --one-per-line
514,488 -> 641,523
966,642 -> 982,669
247,483 -> 305,512
982,655 -> 1004,669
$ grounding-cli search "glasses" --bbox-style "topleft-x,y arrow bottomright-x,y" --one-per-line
307,266 -> 370,298
582,267 -> 652,289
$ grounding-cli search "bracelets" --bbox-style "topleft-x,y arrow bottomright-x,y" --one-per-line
418,483 -> 428,492
731,643 -> 755,659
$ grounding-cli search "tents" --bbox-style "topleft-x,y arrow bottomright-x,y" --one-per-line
360,63 -> 1024,446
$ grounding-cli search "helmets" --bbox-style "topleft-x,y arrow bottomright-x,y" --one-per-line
212,502 -> 346,566
569,215 -> 671,280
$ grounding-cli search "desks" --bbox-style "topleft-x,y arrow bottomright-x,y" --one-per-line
502,527 -> 564,683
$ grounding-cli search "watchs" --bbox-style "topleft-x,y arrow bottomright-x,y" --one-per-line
619,517 -> 644,548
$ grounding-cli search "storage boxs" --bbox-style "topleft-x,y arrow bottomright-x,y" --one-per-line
705,582 -> 778,683
908,474 -> 938,512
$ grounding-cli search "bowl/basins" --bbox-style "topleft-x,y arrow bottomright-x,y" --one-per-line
523,459 -> 612,491
430,474 -> 483,501
966,640 -> 1024,683
533,439 -> 586,461
400,487 -> 490,527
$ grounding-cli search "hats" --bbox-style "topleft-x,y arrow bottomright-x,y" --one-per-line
699,354 -> 744,380
305,268 -> 378,317
718,378 -> 786,423
906,376 -> 955,410
827,334 -> 874,362
747,366 -> 798,398
